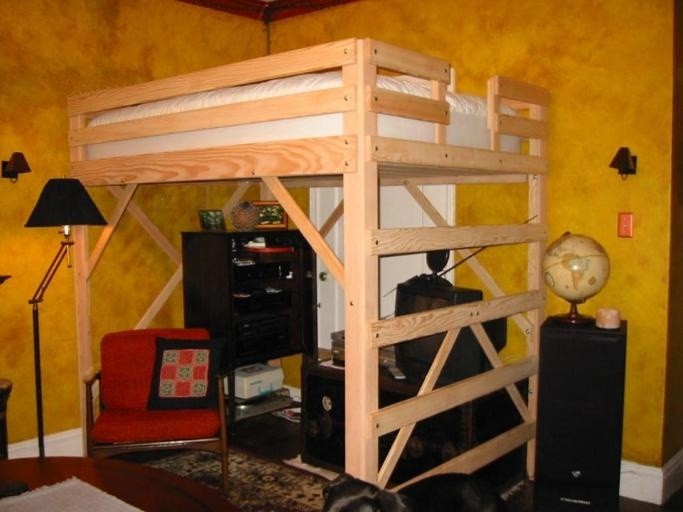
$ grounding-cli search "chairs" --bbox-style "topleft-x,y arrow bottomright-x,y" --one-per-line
83,327 -> 229,496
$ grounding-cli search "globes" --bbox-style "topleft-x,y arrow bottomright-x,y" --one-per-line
542,232 -> 610,325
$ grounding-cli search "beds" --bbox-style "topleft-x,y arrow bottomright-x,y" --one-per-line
68,35 -> 550,502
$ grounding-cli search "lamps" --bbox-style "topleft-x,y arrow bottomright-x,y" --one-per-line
609,147 -> 637,179
25,178 -> 108,462
1,151 -> 31,184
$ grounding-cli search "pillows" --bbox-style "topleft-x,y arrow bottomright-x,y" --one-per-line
146,336 -> 226,410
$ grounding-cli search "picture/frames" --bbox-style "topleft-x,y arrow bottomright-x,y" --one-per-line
197,209 -> 227,232
250,201 -> 289,231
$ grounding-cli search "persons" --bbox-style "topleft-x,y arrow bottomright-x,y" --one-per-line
403,473 -> 509,512
320,473 -> 406,512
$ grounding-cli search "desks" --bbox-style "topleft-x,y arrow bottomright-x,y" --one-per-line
0,457 -> 238,511
299,356 -> 531,512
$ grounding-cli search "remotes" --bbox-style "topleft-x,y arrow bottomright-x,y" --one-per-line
389,364 -> 407,382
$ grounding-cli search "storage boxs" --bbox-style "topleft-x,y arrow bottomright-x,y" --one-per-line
222,365 -> 286,401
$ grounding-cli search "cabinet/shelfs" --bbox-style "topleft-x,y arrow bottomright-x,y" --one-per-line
182,232 -> 318,444
532,318 -> 628,512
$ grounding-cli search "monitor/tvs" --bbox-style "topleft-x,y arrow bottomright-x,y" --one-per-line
395,283 -> 509,385
